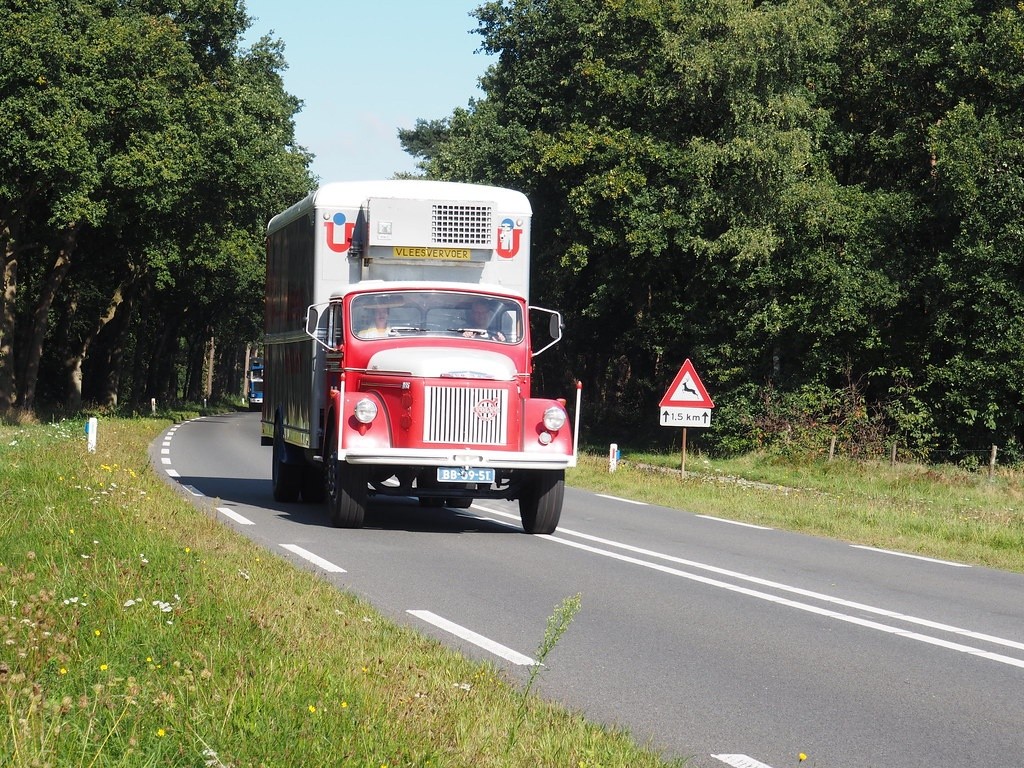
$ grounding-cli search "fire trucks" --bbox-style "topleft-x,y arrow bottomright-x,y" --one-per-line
260,180 -> 583,536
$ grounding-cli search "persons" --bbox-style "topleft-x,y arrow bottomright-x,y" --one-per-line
358,308 -> 401,338
458,298 -> 505,343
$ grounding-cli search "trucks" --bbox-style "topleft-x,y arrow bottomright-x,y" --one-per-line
247,350 -> 264,408
